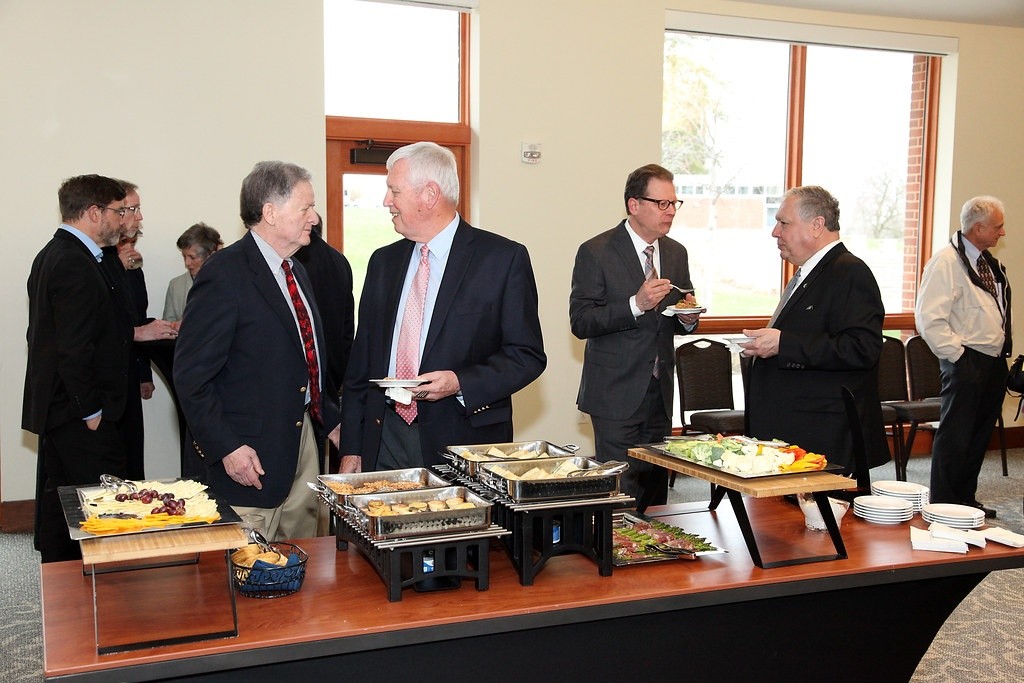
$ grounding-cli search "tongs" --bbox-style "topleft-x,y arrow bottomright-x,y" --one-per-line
642,542 -> 696,560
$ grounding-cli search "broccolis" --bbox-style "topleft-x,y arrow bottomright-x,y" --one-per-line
686,439 -> 798,475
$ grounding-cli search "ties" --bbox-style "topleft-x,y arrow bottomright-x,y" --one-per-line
976,254 -> 997,298
643,246 -> 661,381
281,259 -> 323,422
394,244 -> 430,425
752,268 -> 801,368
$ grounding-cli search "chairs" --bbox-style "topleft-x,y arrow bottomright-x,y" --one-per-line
669,339 -> 746,488
877,334 -> 1008,481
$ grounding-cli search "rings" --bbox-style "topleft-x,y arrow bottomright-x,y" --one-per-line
689,317 -> 692,322
129,258 -> 135,263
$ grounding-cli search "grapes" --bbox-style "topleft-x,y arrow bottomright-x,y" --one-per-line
115,488 -> 187,514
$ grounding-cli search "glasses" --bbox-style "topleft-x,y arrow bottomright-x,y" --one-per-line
124,205 -> 141,212
634,196 -> 684,210
87,204 -> 125,218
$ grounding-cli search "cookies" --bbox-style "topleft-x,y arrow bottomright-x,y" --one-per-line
230,543 -> 288,567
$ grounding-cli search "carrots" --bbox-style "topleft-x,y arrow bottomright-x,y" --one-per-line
78,512 -> 220,535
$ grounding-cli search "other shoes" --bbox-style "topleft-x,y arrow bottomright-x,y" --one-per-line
974,504 -> 997,518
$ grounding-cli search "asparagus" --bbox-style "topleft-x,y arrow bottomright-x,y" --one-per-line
613,520 -> 714,558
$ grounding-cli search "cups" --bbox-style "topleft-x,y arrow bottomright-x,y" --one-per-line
798,474 -> 851,531
234,513 -> 267,552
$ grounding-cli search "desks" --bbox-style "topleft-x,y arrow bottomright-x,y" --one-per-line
43,481 -> 1024,683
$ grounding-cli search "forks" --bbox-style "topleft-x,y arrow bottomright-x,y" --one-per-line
414,391 -> 429,398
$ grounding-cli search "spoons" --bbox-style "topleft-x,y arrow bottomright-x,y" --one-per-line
666,284 -> 695,292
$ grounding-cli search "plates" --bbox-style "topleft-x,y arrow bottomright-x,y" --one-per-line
665,305 -> 705,314
853,480 -> 985,529
370,379 -> 429,387
723,337 -> 757,344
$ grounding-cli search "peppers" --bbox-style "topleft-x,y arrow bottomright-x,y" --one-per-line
757,442 -> 828,472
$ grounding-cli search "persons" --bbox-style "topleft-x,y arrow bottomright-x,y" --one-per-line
162,223 -> 224,324
569,165 -> 700,510
338,141 -> 547,498
172,161 -> 341,548
915,195 -> 1013,519
309,213 -> 324,240
21,176 -> 178,564
739,186 -> 892,508
293,229 -> 355,537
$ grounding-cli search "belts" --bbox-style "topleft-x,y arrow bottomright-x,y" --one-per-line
304,403 -> 310,413
